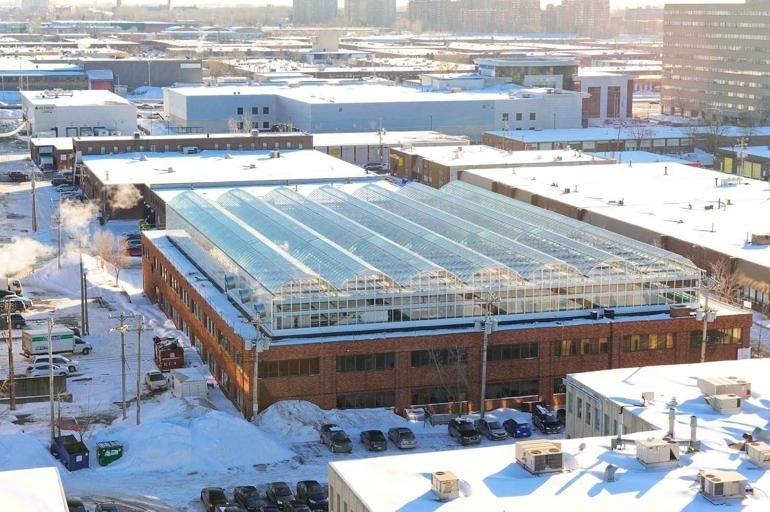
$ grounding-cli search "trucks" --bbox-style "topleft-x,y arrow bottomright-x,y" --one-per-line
0,274 -> 22,295
19,327 -> 92,357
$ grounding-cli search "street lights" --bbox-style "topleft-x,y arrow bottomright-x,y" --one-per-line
696,310 -> 715,363
245,339 -> 269,416
474,320 -> 498,418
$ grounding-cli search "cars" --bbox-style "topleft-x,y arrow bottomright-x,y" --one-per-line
145,370 -> 166,391
51,178 -> 81,200
503,419 -> 531,438
388,428 -> 417,448
360,430 -> 387,451
320,424 -> 352,452
95,504 -> 118,512
448,418 -> 480,444
32,355 -> 80,376
201,480 -> 328,512
67,497 -> 89,512
9,172 -> 28,180
474,418 -> 507,441
0,290 -> 32,330
121,232 -> 141,256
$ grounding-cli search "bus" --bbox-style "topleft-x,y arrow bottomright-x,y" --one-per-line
534,405 -> 561,434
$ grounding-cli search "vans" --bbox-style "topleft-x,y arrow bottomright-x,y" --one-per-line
364,162 -> 382,168
